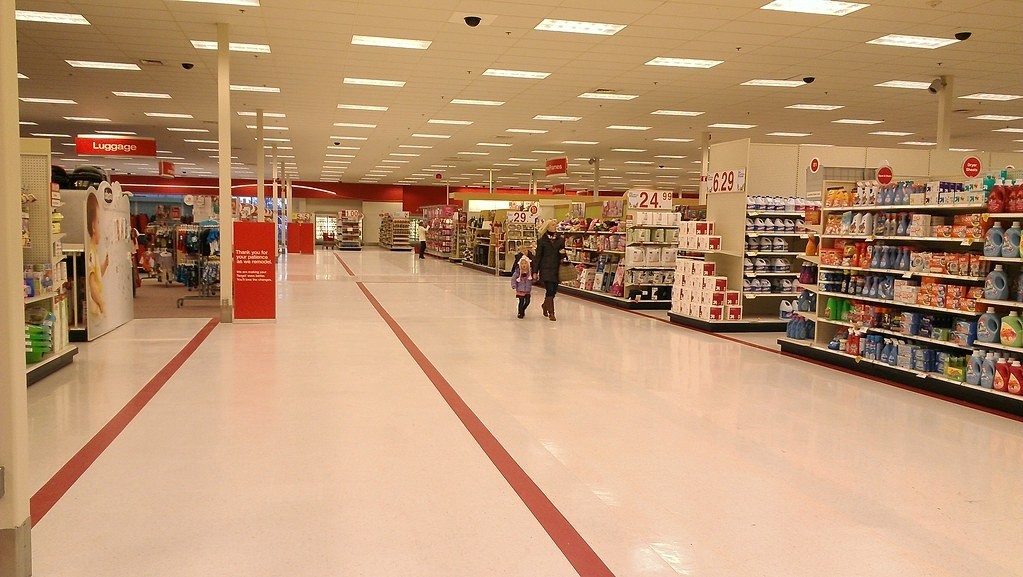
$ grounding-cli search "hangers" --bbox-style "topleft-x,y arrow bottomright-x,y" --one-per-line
146,223 -> 220,267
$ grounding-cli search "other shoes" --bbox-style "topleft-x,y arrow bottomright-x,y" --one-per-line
419,256 -> 425,259
517,312 -> 525,318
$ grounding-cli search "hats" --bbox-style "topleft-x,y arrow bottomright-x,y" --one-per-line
516,255 -> 532,282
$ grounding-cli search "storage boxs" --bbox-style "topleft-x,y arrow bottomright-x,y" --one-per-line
893,177 -> 990,381
628,211 -> 681,285
671,221 -> 742,321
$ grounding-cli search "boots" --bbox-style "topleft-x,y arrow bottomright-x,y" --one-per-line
545,295 -> 556,320
541,296 -> 554,316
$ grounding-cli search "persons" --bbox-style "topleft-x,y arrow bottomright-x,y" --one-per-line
87,193 -> 109,325
950,333 -> 960,343
531,220 -> 567,321
512,255 -> 539,319
511,245 -> 534,277
957,324 -> 962,331
418,222 -> 430,259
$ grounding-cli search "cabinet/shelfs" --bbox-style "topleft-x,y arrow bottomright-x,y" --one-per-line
378,204 -> 537,278
776,179 -> 1023,418
532,225 -> 679,310
667,193 -> 821,333
336,219 -> 363,251
18,138 -> 87,390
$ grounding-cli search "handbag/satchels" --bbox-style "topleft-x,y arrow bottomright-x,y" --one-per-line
558,252 -> 577,281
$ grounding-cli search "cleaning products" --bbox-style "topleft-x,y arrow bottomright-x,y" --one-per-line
742,178 -> 1023,397
